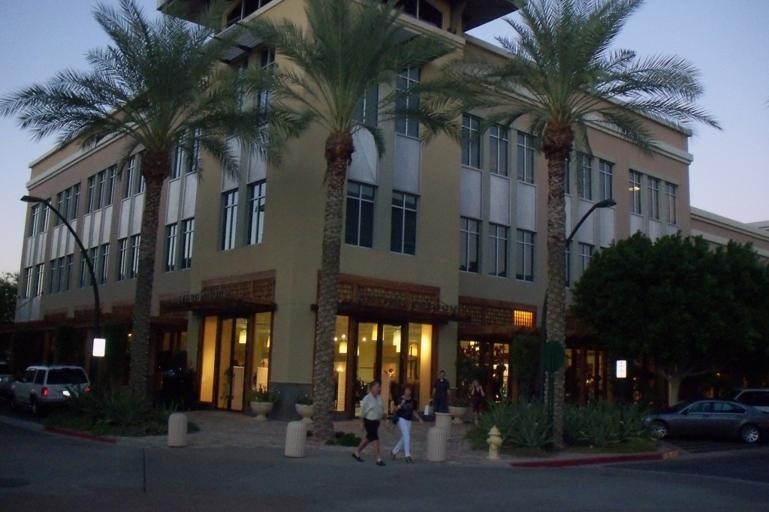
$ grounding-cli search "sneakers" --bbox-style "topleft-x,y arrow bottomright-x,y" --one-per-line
389,449 -> 415,463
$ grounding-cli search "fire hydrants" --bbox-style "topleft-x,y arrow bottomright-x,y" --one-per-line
485,424 -> 505,461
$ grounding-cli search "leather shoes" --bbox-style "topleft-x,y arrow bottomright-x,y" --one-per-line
351,452 -> 364,462
376,460 -> 386,466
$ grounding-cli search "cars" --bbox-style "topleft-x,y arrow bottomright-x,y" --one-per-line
645,388 -> 769,445
1,364 -> 93,414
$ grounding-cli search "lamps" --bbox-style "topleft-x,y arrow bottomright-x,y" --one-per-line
338,334 -> 348,353
372,323 -> 385,342
393,325 -> 402,352
408,342 -> 418,361
238,325 -> 247,345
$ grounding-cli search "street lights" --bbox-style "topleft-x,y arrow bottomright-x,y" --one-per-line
21,195 -> 102,429
537,198 -> 617,412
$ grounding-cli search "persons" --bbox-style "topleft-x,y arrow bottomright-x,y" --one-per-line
470,379 -> 486,423
353,380 -> 387,467
431,369 -> 449,413
391,384 -> 426,463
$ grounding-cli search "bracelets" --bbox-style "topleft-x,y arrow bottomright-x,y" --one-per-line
398,405 -> 401,409
417,416 -> 421,420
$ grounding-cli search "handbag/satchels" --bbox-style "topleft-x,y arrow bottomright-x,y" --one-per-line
392,410 -> 400,424
423,399 -> 435,415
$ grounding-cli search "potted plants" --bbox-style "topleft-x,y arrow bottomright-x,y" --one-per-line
294,392 -> 315,425
511,394 -> 556,457
246,387 -> 281,423
447,398 -> 470,425
434,407 -> 453,442
